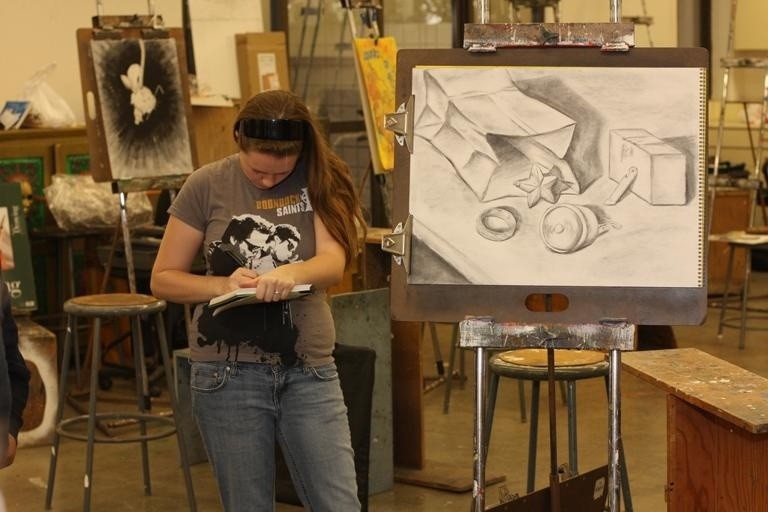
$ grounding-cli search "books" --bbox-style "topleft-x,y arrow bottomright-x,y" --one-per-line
210,283 -> 313,315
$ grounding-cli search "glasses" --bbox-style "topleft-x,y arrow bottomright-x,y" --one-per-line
286,240 -> 295,254
244,240 -> 263,254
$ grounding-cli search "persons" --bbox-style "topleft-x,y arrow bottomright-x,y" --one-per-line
0,276 -> 30,469
149,90 -> 369,511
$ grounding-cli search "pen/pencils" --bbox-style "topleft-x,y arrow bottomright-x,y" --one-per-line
225,250 -> 247,269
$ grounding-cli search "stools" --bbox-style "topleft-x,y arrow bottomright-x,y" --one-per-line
717,231 -> 768,349
484,347 -> 634,512
44,294 -> 198,511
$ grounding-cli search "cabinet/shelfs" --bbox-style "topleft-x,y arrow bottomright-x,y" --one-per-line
0,126 -> 90,334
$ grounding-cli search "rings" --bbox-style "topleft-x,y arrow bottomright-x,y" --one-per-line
274,290 -> 282,295
